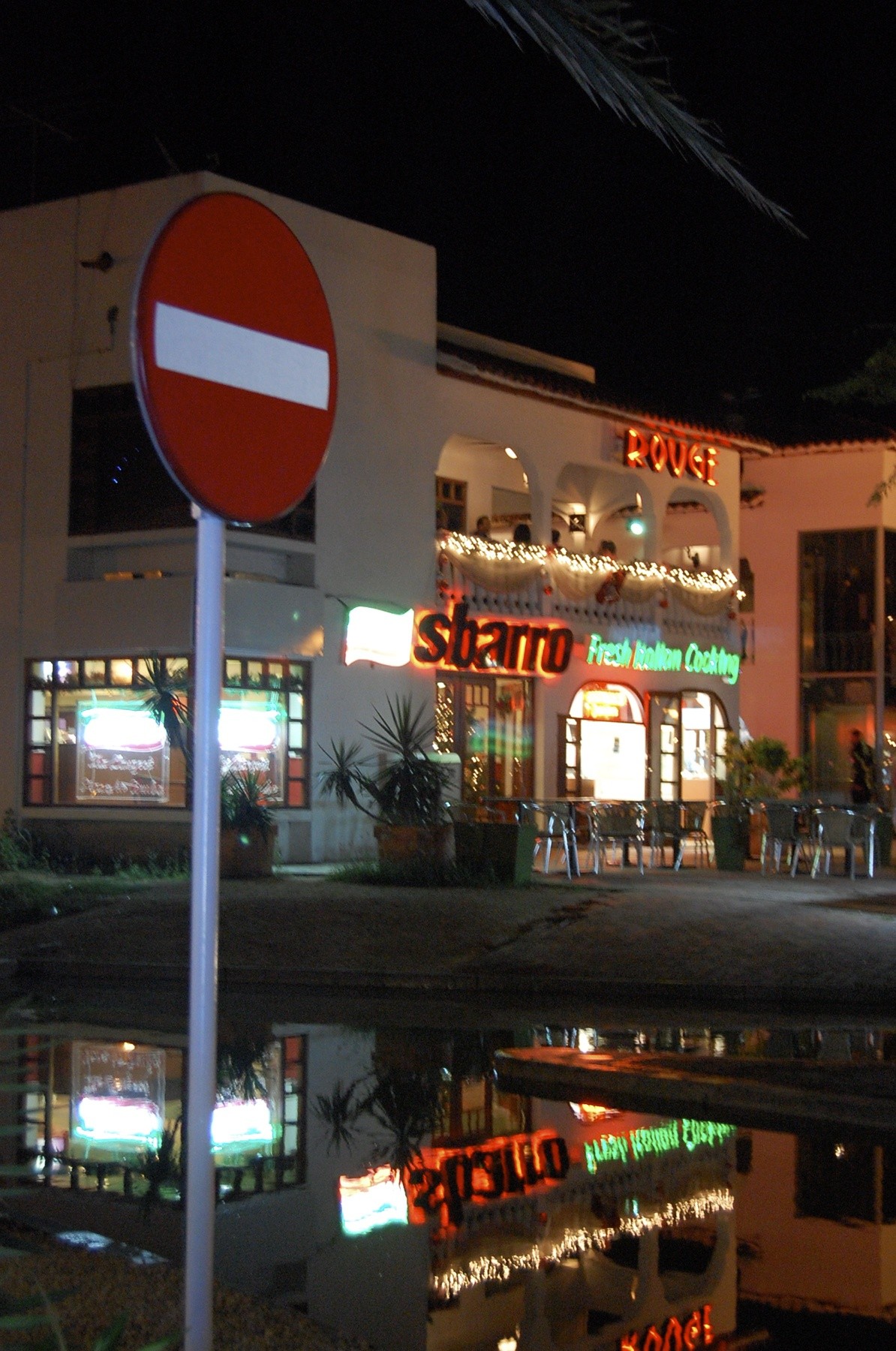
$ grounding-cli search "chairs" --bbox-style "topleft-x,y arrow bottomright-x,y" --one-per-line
442,800 -> 522,826
519,797 -> 580,880
576,799 -> 648,876
808,806 -> 873,881
757,801 -> 811,879
647,799 -> 714,873
658,802 -> 711,868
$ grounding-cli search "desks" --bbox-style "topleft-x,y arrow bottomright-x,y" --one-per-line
785,803 -> 896,872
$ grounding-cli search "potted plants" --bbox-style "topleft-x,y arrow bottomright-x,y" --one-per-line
122,647 -> 280,881
311,687 -> 459,884
711,732 -> 814,873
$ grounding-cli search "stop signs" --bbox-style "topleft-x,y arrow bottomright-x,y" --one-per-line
134,189 -> 339,528
